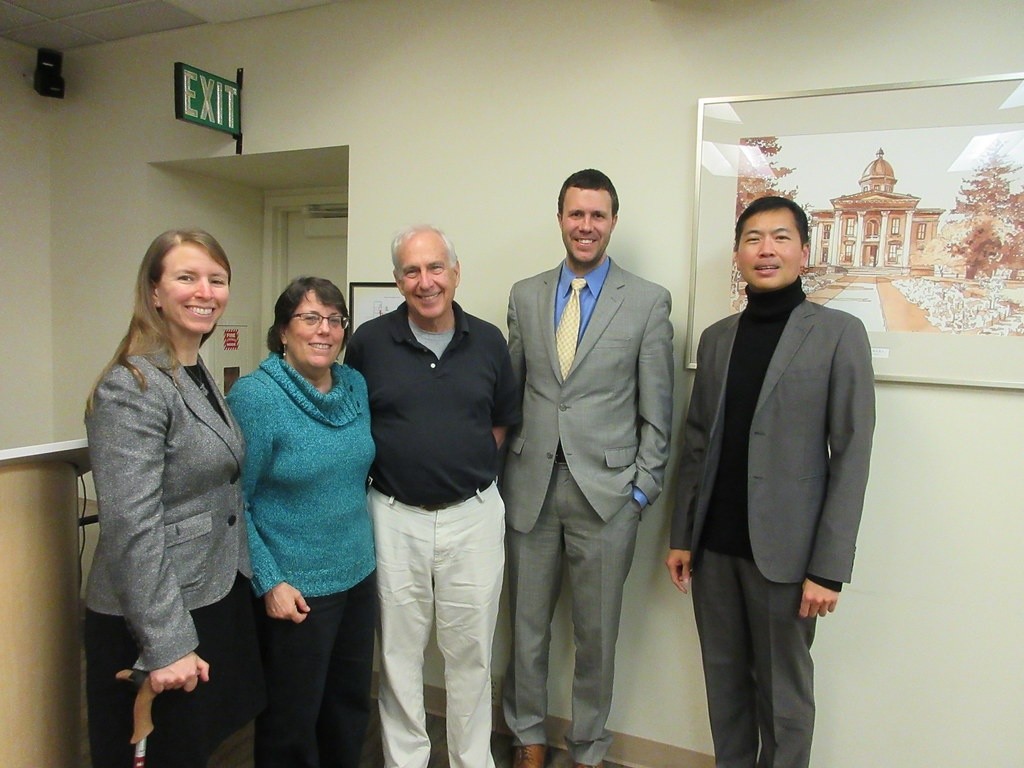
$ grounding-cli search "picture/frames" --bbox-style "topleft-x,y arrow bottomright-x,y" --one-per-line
682,71 -> 1024,389
347,282 -> 406,340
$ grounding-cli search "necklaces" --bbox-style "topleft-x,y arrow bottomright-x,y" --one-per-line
180,357 -> 210,398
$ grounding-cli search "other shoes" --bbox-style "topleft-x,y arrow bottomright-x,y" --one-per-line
572,760 -> 603,768
513,744 -> 547,768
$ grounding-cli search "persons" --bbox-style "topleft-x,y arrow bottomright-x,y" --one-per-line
339,226 -> 520,767
665,195 -> 876,766
81,227 -> 257,767
218,277 -> 379,766
505,168 -> 674,768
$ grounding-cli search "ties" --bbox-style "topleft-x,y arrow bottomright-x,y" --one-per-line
555,278 -> 586,382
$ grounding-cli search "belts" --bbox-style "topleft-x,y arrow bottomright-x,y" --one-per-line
414,502 -> 450,511
554,455 -> 566,464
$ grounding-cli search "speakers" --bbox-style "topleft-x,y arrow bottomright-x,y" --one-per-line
32,47 -> 65,99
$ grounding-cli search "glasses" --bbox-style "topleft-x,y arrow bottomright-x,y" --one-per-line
290,313 -> 351,331
